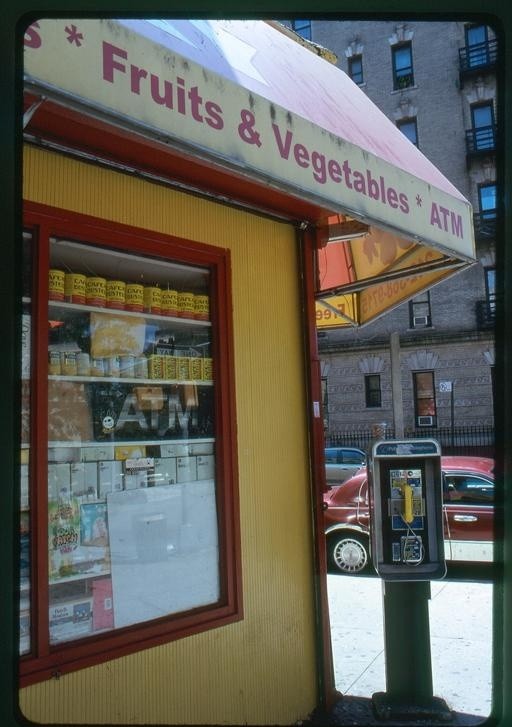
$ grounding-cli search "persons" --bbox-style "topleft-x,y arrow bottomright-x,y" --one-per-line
369,428 -> 384,458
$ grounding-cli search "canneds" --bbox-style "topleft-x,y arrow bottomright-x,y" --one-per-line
49,350 -> 149,379
49,269 -> 211,321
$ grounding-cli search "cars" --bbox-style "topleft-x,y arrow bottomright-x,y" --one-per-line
323,455 -> 495,574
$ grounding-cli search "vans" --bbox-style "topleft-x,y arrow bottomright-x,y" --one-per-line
324,447 -> 367,490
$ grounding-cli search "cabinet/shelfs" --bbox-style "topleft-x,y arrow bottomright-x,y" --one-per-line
18,230 -> 222,656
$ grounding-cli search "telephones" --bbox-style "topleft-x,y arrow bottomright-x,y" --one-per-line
390,469 -> 425,531
